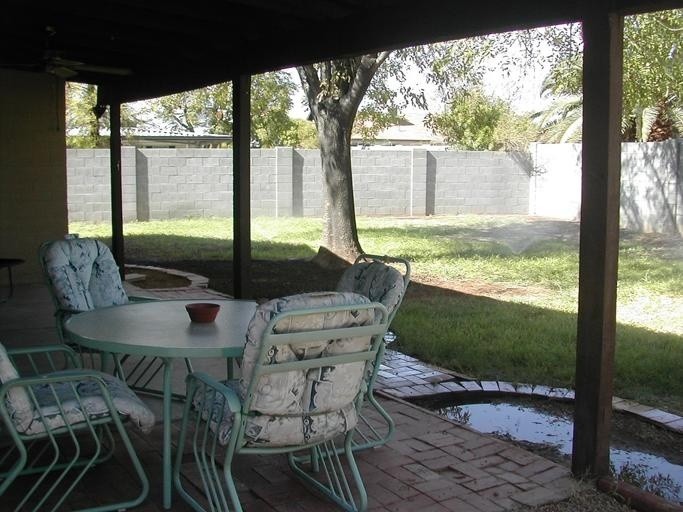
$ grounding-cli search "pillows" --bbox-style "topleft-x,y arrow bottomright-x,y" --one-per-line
314,246 -> 353,269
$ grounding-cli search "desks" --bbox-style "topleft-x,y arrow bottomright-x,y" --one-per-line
65,300 -> 260,510
2,259 -> 24,302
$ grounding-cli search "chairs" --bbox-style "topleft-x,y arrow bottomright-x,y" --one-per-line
1,345 -> 155,511
41,237 -> 192,404
175,291 -> 389,511
235,254 -> 412,463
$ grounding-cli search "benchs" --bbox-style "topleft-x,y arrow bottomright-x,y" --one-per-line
208,258 -> 350,306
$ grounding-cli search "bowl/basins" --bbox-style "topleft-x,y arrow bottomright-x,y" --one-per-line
184,303 -> 220,323
